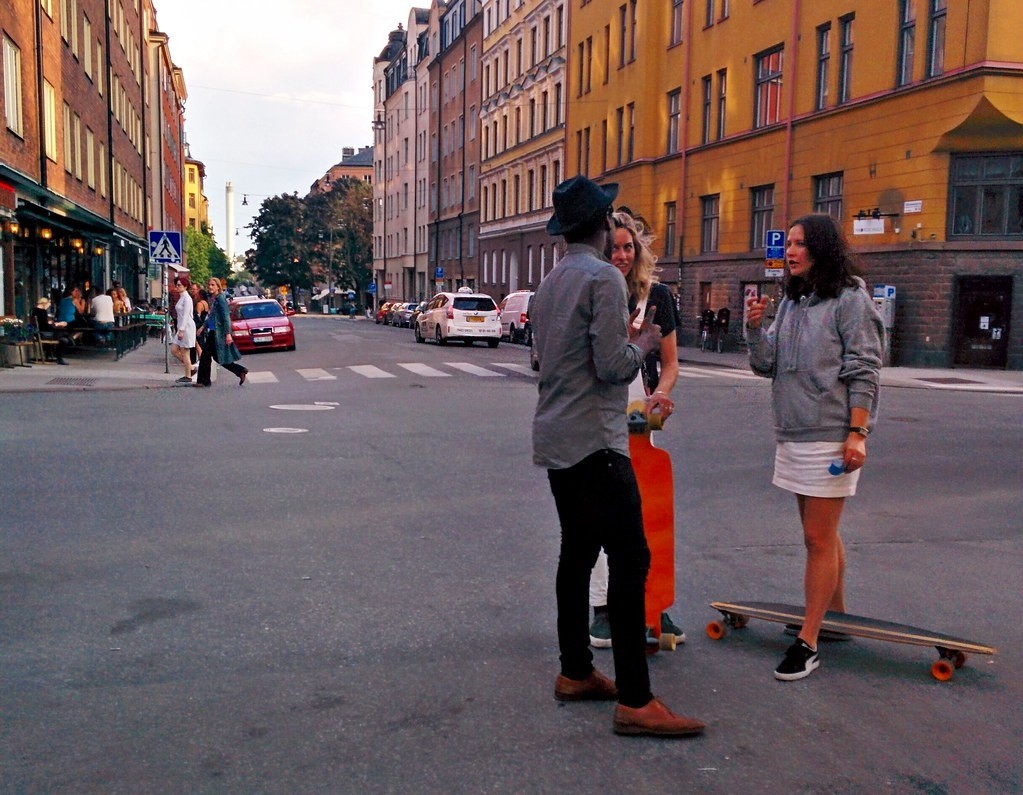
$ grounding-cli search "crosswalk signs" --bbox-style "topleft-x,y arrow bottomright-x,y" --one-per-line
148,230 -> 183,264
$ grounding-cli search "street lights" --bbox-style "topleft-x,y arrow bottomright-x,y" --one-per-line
318,230 -> 332,314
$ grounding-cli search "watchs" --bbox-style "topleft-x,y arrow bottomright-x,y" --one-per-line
849,427 -> 870,438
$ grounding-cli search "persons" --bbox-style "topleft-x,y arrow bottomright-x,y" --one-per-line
168,276 -> 211,383
530,175 -> 707,736
192,277 -> 249,387
589,212 -> 686,648
33,280 -> 158,365
744,212 -> 887,681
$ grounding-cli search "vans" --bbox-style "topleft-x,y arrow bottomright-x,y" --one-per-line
498,291 -> 535,342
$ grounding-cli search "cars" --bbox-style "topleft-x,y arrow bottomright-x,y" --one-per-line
414,286 -> 502,348
227,295 -> 258,318
285,301 -> 307,313
409,300 -> 424,329
231,298 -> 297,353
375,302 -> 419,328
524,312 -> 540,371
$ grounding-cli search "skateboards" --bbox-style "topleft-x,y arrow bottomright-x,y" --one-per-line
706,602 -> 995,680
626,399 -> 677,654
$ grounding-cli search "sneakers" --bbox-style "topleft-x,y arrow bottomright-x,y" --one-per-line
648,614 -> 684,644
589,614 -> 612,648
774,636 -> 819,681
783,621 -> 854,640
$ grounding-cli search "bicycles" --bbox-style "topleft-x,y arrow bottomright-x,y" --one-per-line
698,309 -> 730,352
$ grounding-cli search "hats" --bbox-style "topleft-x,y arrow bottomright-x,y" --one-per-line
545,175 -> 621,239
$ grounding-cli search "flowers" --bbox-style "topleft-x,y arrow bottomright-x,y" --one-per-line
0,318 -> 38,342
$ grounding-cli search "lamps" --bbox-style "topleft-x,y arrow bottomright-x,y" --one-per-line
8,218 -> 104,255
853,208 -> 899,219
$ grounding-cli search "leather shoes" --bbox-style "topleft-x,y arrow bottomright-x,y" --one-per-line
553,667 -> 619,702
612,696 -> 705,736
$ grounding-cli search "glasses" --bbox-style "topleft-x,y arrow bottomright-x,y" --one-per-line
175,284 -> 182,287
604,205 -> 614,214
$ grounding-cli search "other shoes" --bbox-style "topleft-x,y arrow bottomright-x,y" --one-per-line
57,358 -> 69,365
192,381 -> 207,386
238,369 -> 248,385
176,376 -> 192,383
190,368 -> 198,376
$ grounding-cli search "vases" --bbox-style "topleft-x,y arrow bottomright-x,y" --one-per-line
0,340 -> 42,366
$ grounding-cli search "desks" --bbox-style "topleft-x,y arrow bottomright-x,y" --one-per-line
75,310 -> 172,350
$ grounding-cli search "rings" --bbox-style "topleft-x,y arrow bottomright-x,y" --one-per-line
851,457 -> 858,462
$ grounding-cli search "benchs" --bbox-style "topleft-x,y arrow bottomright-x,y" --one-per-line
21,322 -> 172,360
